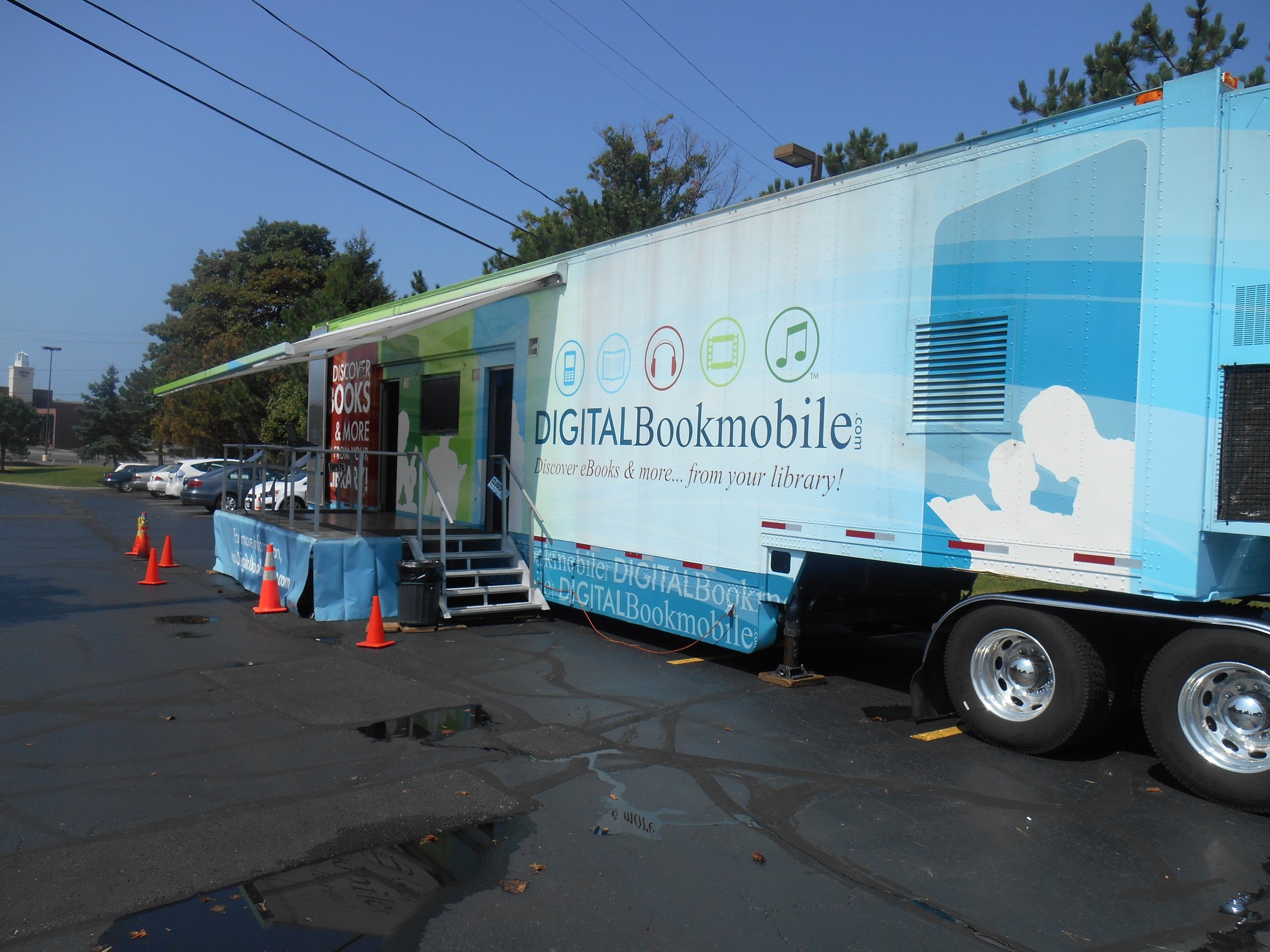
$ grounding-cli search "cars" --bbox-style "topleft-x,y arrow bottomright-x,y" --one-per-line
102,458 -> 308,511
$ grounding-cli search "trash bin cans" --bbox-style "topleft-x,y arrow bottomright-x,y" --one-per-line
396,559 -> 445,627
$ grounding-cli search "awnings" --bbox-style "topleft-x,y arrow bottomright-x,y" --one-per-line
150,273 -> 566,398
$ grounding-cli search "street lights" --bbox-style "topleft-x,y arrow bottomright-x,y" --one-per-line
43,346 -> 62,462
773,142 -> 823,183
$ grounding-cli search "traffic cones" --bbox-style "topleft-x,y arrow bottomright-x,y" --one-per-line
131,531 -> 150,561
158,535 -> 179,567
137,548 -> 167,585
355,594 -> 398,648
252,544 -> 288,614
124,512 -> 151,555
